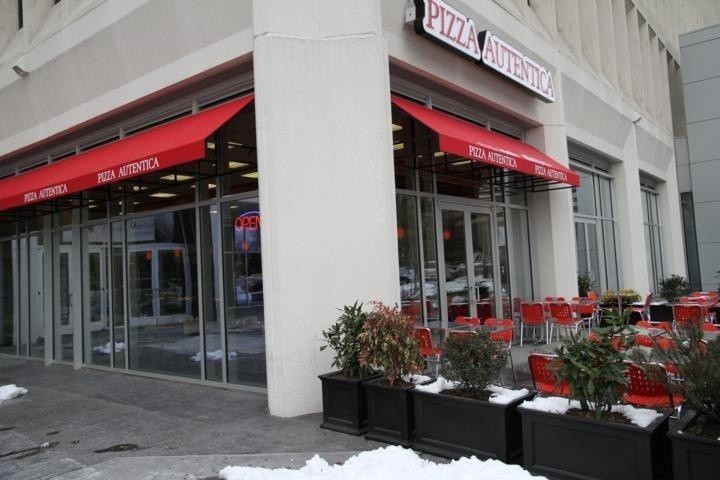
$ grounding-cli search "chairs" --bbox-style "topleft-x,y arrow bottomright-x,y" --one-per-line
413,314 -> 515,384
520,290 -> 720,396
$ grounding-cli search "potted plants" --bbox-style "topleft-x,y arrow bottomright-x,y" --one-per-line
412,325 -> 530,463
517,303 -> 676,478
317,300 -> 378,437
632,316 -> 720,479
354,302 -> 422,446
